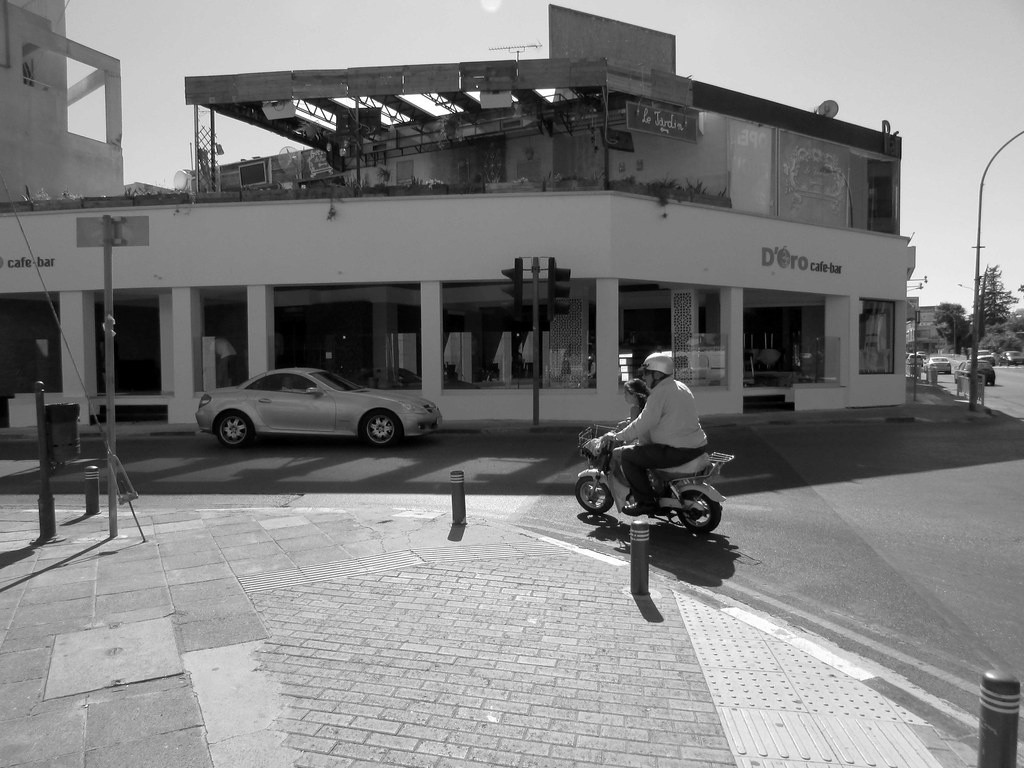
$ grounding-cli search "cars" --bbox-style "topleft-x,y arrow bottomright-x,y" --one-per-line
344,367 -> 421,389
954,360 -> 996,386
998,351 -> 1024,367
968,349 -> 996,366
906,350 -> 927,368
195,366 -> 442,451
924,357 -> 951,374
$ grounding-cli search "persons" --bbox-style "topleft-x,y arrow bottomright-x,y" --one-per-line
561,356 -> 572,383
215,338 -> 237,387
601,352 -> 708,516
587,356 -> 596,379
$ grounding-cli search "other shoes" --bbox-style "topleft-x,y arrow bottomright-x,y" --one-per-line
622,500 -> 657,517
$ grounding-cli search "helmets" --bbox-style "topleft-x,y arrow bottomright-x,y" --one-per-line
643,352 -> 674,375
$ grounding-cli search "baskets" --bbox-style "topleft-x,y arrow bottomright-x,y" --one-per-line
577,424 -> 615,459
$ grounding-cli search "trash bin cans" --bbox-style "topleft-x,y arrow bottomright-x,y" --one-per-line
927,365 -> 938,385
46,402 -> 82,462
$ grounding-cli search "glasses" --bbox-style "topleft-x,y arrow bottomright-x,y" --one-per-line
644,371 -> 655,376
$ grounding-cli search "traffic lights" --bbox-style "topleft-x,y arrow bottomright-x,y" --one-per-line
547,257 -> 572,322
499,257 -> 524,326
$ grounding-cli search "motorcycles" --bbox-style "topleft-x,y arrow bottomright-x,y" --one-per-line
575,418 -> 736,535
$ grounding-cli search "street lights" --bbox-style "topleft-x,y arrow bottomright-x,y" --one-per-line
934,308 -> 957,360
